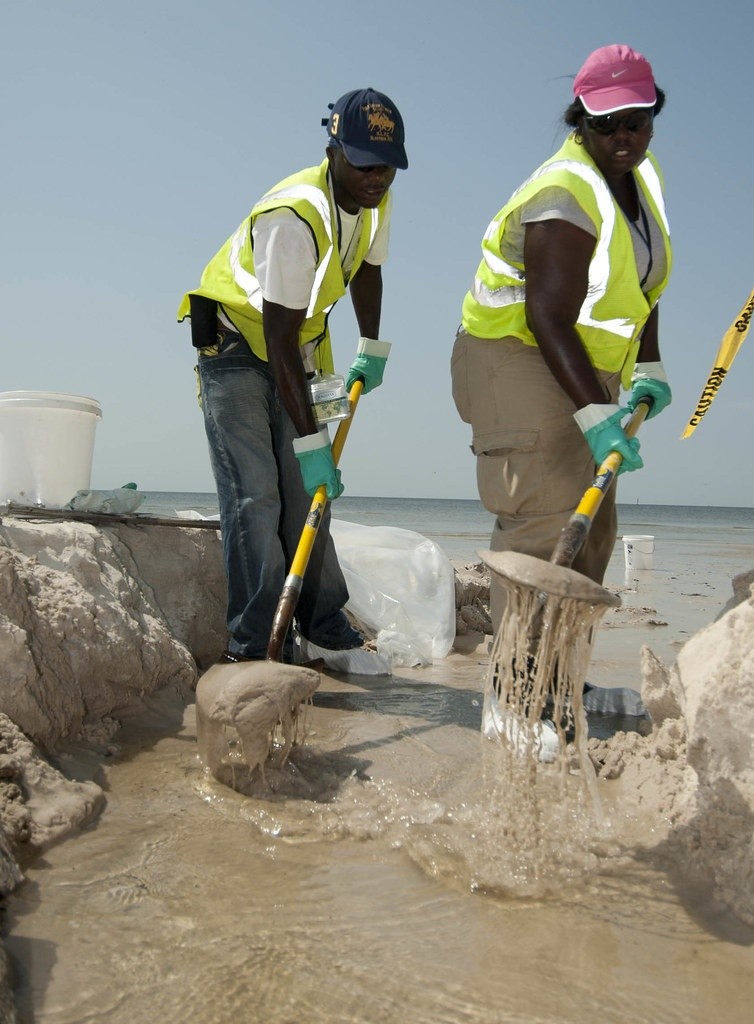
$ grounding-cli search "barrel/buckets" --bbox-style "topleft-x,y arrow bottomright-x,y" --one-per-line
621,535 -> 655,571
0,391 -> 101,510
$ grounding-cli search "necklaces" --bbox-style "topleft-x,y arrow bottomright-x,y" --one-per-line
611,190 -> 654,289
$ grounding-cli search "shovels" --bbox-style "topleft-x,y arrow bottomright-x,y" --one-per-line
475,394 -> 654,604
196,375 -> 365,727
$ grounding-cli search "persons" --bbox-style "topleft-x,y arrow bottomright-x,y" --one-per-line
451,45 -> 672,744
179,87 -> 409,677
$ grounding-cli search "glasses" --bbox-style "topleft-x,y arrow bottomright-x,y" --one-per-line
584,106 -> 656,135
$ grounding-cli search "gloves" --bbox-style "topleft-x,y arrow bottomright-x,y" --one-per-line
344,338 -> 391,395
292,427 -> 344,502
627,360 -> 672,421
573,403 -> 644,477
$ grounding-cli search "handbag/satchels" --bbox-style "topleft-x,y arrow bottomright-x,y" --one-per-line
189,294 -> 218,347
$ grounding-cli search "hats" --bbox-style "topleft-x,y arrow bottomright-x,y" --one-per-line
320,88 -> 408,170
572,43 -> 658,116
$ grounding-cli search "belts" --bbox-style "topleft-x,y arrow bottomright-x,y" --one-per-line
217,320 -> 229,331
